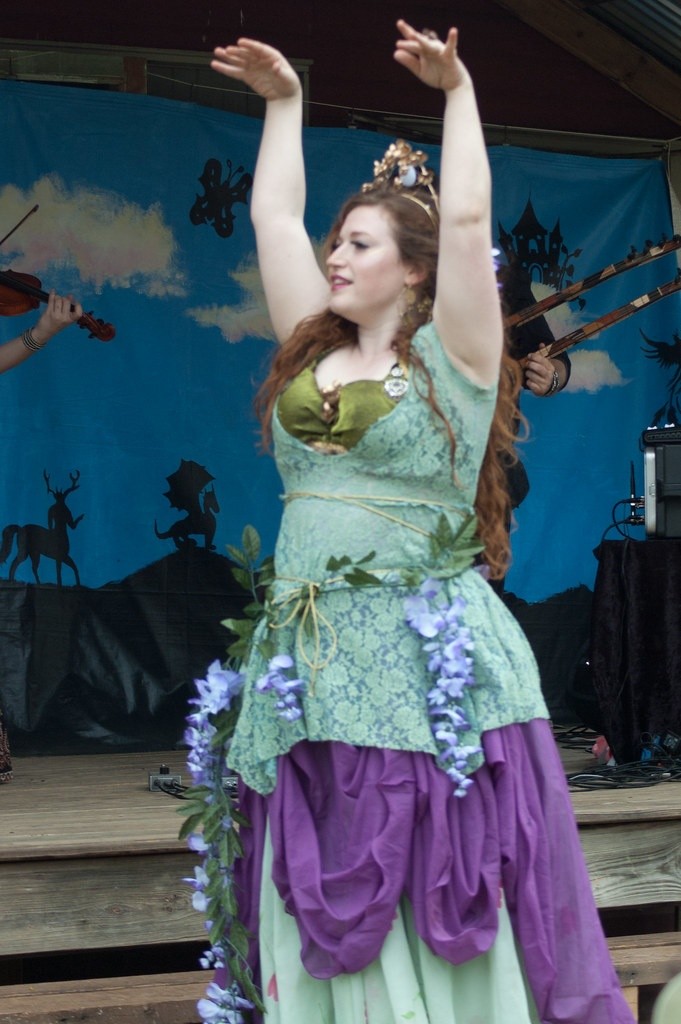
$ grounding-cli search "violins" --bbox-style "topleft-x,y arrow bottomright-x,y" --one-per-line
1,266 -> 120,343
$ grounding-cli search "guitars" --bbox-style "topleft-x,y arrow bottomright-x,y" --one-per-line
508,226 -> 680,366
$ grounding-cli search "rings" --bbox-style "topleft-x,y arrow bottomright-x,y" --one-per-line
422,28 -> 438,40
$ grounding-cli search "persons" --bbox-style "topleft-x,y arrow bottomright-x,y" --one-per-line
468,262 -> 573,601
210,17 -> 636,1024
0,289 -> 82,372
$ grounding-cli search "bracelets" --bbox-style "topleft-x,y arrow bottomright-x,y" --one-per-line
544,370 -> 560,398
22,325 -> 47,352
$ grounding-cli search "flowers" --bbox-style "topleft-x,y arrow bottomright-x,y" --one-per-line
175,511 -> 489,1024
